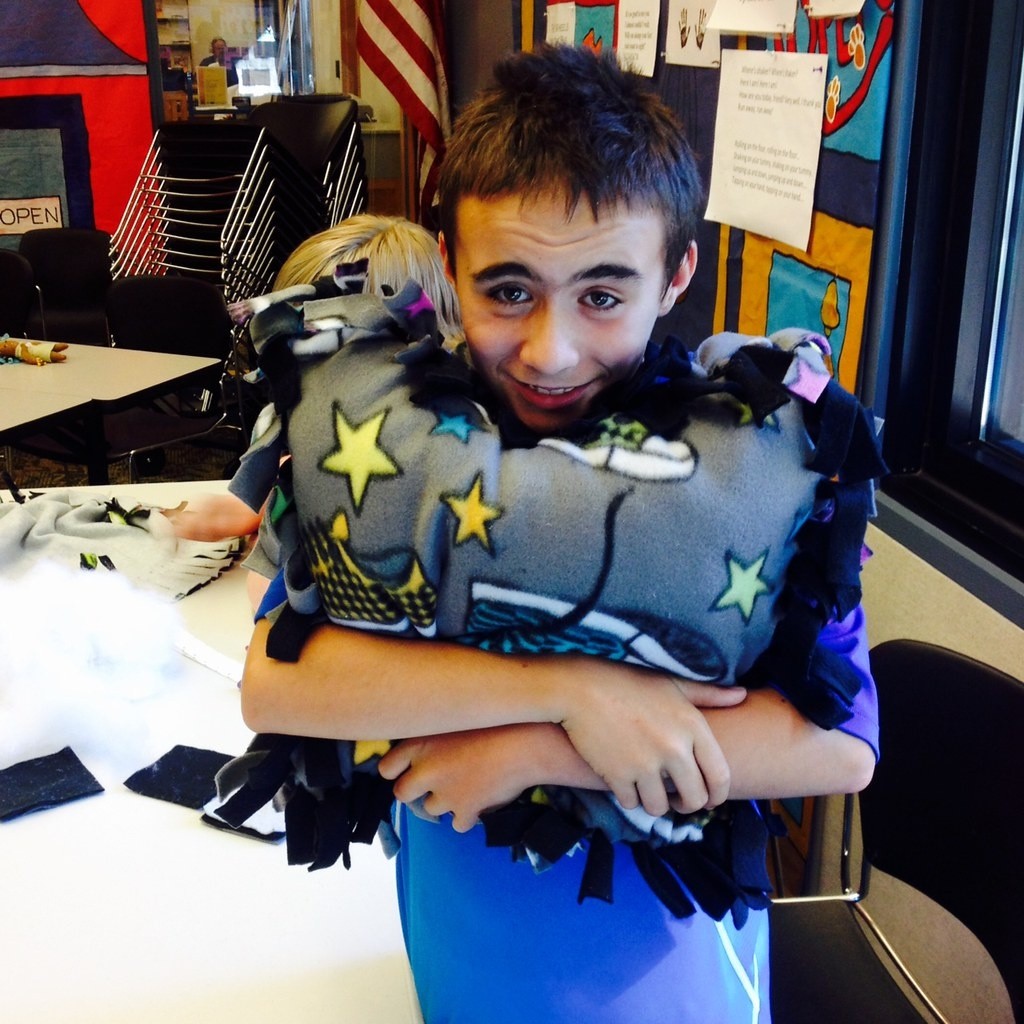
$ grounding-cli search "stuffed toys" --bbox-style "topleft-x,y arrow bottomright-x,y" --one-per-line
0,340 -> 69,367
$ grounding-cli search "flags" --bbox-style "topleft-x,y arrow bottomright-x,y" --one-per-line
357,0 -> 450,232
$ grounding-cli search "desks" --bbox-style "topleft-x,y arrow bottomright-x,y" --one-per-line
0,333 -> 224,488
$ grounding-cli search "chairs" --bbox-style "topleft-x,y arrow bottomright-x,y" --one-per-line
0,94 -> 370,479
770,636 -> 1024,1024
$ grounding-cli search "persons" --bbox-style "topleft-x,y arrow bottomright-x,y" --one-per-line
199,38 -> 239,88
174,213 -> 463,620
242,37 -> 877,1024
